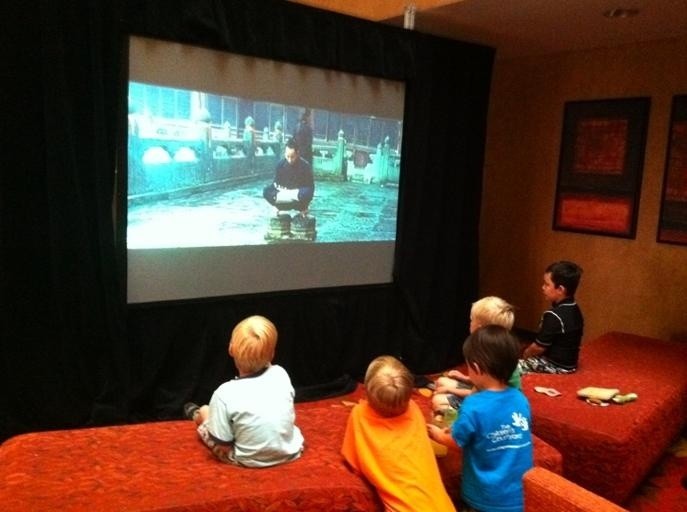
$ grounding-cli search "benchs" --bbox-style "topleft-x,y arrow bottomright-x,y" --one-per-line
420,330 -> 687,512
3,377 -> 565,512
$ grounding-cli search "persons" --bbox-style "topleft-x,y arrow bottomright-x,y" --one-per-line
430,294 -> 523,416
261,137 -> 316,217
288,105 -> 314,166
426,322 -> 534,511
340,355 -> 456,511
182,314 -> 306,468
517,260 -> 584,374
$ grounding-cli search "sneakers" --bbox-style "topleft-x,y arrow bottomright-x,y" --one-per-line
184,401 -> 202,420
